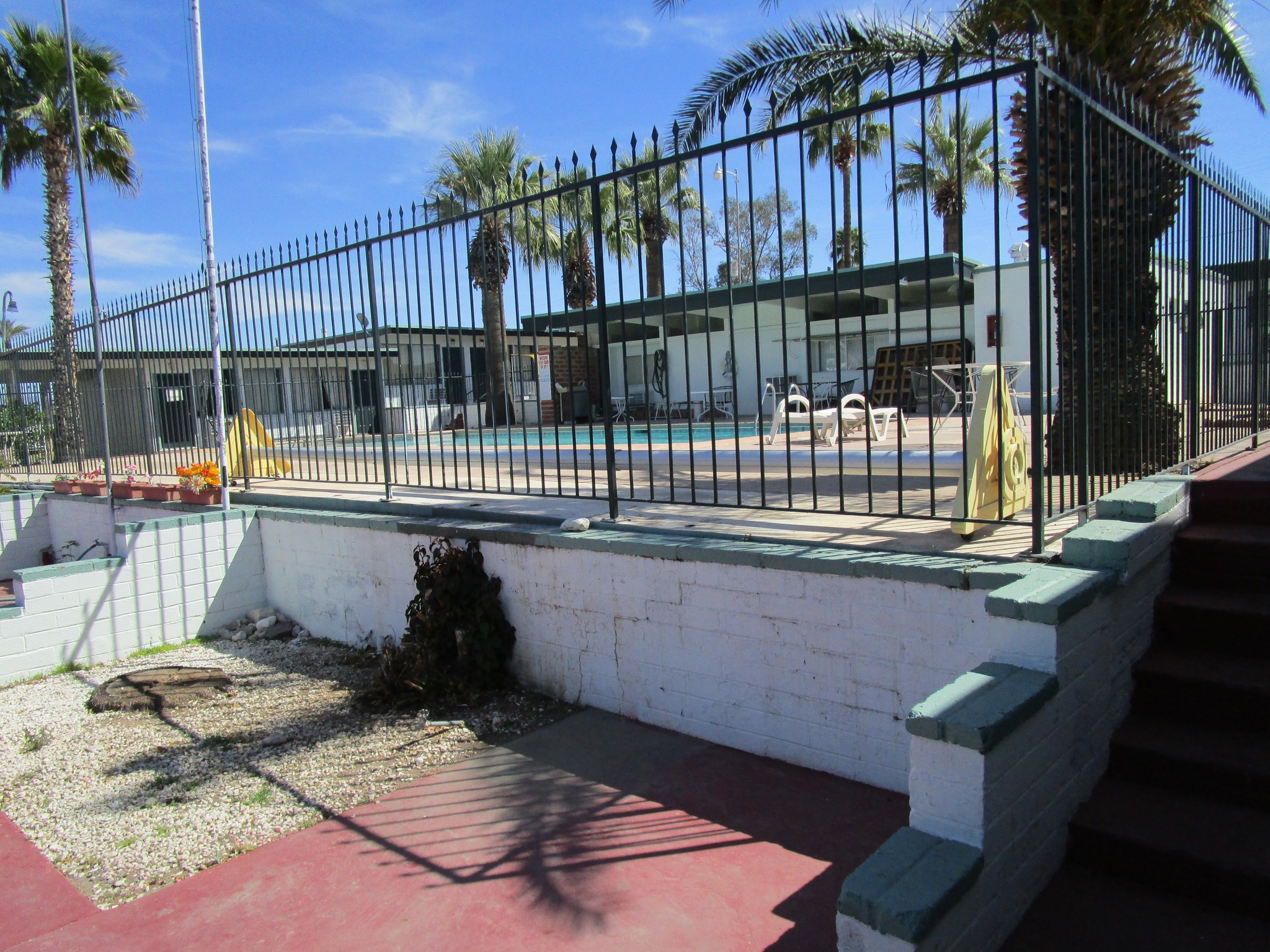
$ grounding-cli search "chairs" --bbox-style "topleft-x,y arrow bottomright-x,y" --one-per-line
706,386 -> 734,420
903,357 -> 969,430
809,379 -> 830,411
966,365 -> 1027,426
827,378 -> 859,409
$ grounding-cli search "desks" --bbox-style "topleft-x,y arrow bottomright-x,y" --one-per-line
611,396 -> 635,423
685,390 -> 735,423
925,361 -> 1031,441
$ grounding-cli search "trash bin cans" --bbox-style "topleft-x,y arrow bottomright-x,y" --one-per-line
557,386 -> 589,422
693,400 -> 704,422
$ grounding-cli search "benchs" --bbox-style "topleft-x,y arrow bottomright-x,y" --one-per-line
766,394 -> 909,446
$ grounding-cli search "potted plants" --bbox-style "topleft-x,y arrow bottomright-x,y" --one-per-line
139,466 -> 180,501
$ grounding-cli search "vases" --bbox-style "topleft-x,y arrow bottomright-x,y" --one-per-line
176,486 -> 222,505
51,481 -> 83,494
112,482 -> 147,498
77,481 -> 107,496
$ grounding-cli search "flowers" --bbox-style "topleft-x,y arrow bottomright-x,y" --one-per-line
82,461 -> 105,482
118,464 -> 150,483
54,471 -> 81,481
175,461 -> 222,494
